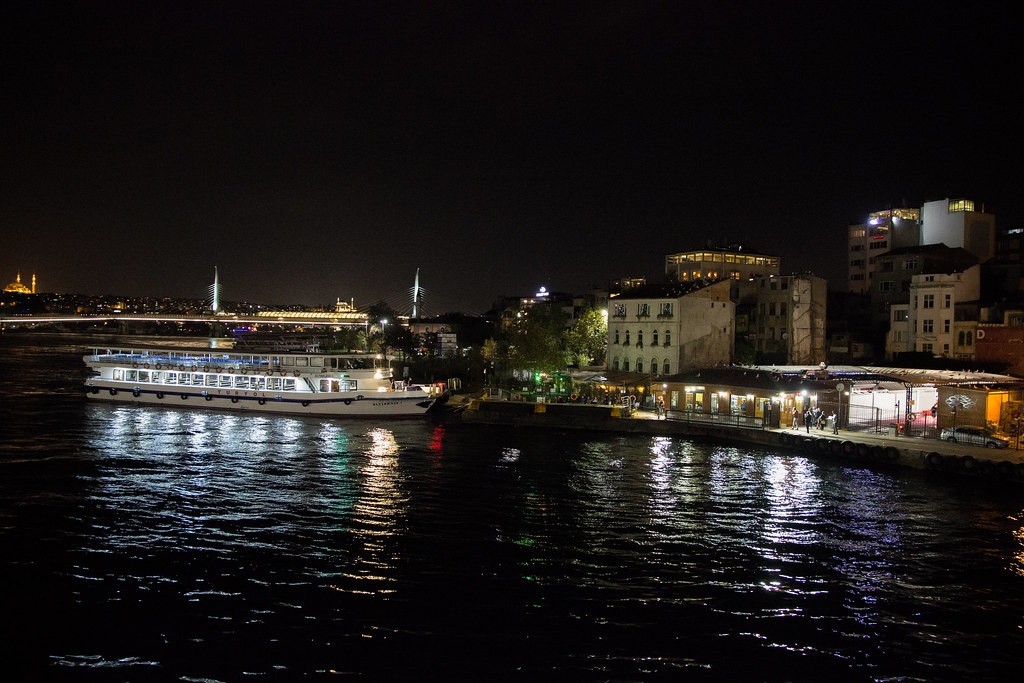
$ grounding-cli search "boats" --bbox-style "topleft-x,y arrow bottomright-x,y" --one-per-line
83,367 -> 438,414
83,348 -> 394,379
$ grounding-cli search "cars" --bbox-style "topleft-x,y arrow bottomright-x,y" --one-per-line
941,425 -> 1010,448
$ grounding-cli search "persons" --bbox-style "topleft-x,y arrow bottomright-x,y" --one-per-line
660,397 -> 665,407
741,400 -> 746,411
792,407 -> 799,429
831,410 -> 839,435
804,401 -> 826,433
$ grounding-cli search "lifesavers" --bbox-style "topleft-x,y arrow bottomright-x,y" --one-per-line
204,365 -> 209,372
514,392 -> 520,400
144,363 -> 150,369
241,367 -> 248,374
266,369 -> 274,376
216,366 -> 222,373
191,364 -> 198,371
254,368 -> 260,375
293,369 -> 301,376
167,364 -> 174,370
132,362 -> 139,368
228,367 -> 234,373
907,413 -> 916,422
571,393 -> 578,400
155,363 -> 161,369
179,364 -> 185,371
84,388 -> 352,408
280,369 -> 287,377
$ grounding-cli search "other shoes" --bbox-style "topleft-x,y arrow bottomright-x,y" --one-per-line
790,428 -> 794,430
795,428 -> 798,430
831,432 -> 839,435
